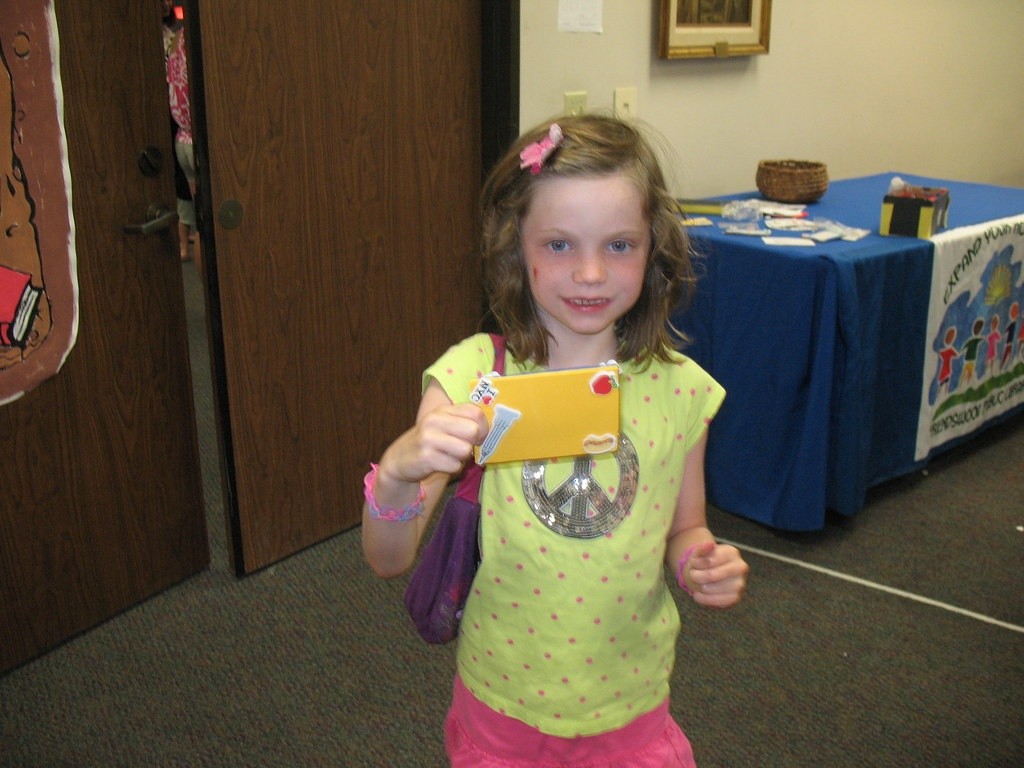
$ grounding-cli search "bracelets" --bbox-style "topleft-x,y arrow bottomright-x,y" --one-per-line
363,462 -> 427,522
676,543 -> 701,598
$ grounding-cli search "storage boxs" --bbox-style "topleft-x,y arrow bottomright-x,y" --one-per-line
880,190 -> 950,241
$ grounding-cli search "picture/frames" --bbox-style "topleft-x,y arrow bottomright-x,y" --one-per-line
656,0 -> 772,61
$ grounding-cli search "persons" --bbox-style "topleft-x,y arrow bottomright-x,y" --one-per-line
360,110 -> 749,768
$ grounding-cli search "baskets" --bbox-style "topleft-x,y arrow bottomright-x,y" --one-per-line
755,160 -> 830,204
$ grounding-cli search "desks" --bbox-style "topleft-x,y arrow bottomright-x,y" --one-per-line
668,172 -> 1024,534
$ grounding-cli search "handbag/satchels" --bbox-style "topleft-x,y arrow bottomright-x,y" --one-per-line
402,464 -> 490,646
